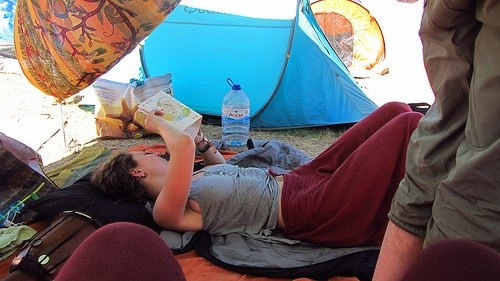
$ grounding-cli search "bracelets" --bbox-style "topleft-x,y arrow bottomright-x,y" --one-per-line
196,135 -> 217,154
198,143 -> 210,153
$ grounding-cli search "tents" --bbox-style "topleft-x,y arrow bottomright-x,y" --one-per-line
310,0 -> 388,79
139,0 -> 379,129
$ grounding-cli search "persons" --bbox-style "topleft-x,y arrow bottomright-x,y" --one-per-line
370,0 -> 500,281
90,101 -> 424,247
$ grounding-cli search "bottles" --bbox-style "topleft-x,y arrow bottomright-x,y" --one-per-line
222,76 -> 251,146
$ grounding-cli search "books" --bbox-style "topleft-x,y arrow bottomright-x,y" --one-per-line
138,91 -> 202,140
